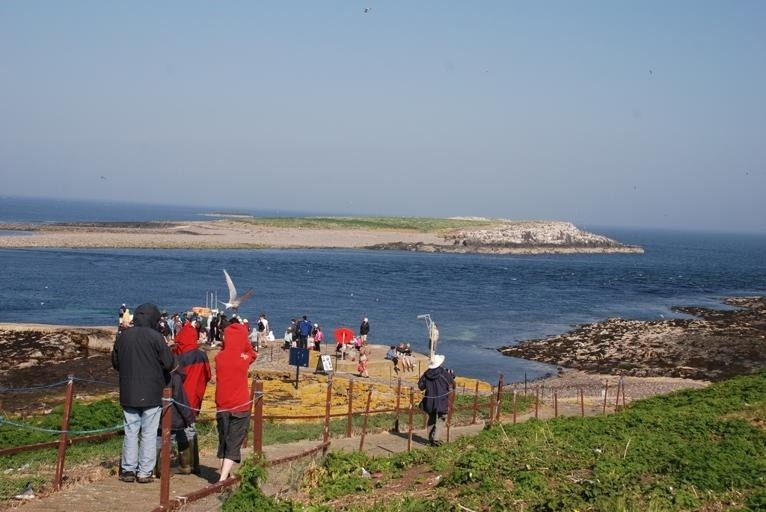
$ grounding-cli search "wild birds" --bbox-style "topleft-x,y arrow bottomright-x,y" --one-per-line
221,268 -> 254,314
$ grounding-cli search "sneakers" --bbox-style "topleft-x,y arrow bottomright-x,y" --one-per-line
119,471 -> 135,482
138,470 -> 154,483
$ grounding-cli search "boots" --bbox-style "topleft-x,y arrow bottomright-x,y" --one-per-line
170,446 -> 192,475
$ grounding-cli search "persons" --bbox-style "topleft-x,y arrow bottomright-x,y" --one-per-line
214,323 -> 257,484
115,302 -> 419,379
111,302 -> 176,484
170,322 -> 211,474
417,354 -> 454,447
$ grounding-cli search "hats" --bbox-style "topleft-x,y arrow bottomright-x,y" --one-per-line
428,354 -> 445,369
